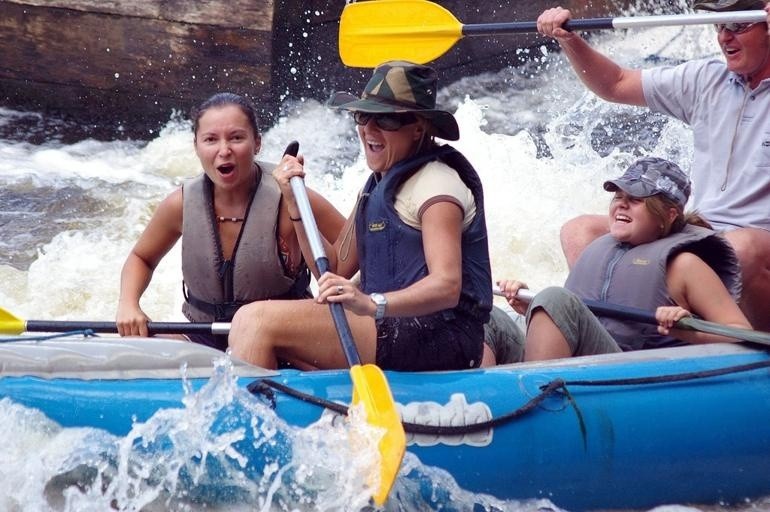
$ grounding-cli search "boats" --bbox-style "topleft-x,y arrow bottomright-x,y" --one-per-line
0,302 -> 768,507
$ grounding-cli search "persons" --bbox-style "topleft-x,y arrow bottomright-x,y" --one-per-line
481,158 -> 754,364
536,0 -> 770,331
116,93 -> 348,352
229,61 -> 493,371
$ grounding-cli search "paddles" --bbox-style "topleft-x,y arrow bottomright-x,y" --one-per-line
1,308 -> 231,339
282,140 -> 408,505
339,1 -> 768,69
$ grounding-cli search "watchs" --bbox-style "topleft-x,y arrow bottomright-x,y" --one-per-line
370,292 -> 387,322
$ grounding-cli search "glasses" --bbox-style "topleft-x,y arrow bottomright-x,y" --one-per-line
353,110 -> 426,131
714,22 -> 752,32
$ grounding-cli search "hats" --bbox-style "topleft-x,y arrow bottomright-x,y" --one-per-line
693,0 -> 767,11
327,60 -> 460,140
603,157 -> 691,209
